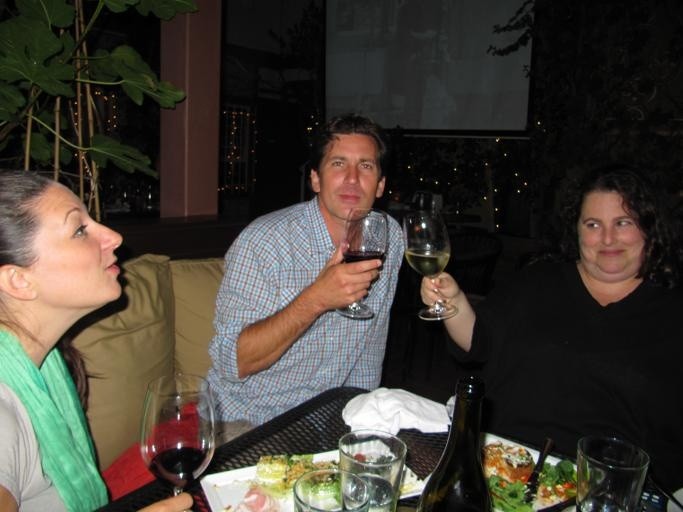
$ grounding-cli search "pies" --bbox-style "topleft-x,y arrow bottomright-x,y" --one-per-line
483,444 -> 535,484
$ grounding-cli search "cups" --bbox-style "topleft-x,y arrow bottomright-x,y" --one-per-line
573,435 -> 651,512
338,429 -> 408,511
291,469 -> 372,512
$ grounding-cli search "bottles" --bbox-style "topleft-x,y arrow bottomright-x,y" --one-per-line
415,374 -> 495,512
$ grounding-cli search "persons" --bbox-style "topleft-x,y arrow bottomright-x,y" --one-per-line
198,114 -> 406,466
0,168 -> 196,512
420,168 -> 682,504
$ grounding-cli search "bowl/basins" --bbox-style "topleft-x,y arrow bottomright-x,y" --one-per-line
666,488 -> 683,512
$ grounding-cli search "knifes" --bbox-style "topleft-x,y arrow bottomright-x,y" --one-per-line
523,435 -> 557,502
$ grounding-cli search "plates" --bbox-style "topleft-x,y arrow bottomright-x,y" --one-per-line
199,439 -> 425,512
423,430 -> 596,512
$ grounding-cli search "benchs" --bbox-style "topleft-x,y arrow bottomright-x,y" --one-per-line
63,214 -> 251,503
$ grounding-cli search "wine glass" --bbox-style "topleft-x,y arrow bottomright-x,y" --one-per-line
402,207 -> 458,321
139,373 -> 217,497
335,208 -> 389,320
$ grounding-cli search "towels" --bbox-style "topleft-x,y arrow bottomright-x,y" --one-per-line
337,385 -> 448,439
